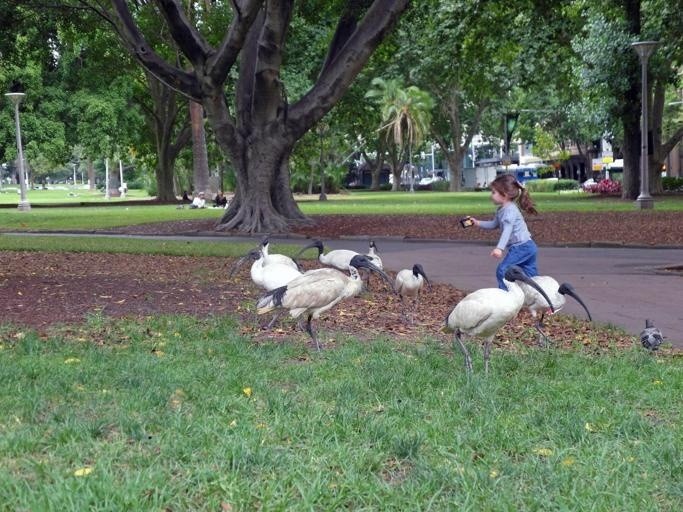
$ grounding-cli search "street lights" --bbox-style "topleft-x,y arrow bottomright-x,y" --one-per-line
316,121 -> 327,201
3,91 -> 33,210
22,151 -> 29,191
628,39 -> 662,211
72,163 -> 76,184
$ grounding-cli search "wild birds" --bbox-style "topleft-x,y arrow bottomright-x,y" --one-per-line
514,274 -> 592,350
255,255 -> 394,353
255,235 -> 299,272
444,266 -> 553,373
293,239 -> 394,292
394,263 -> 431,326
366,239 -> 384,289
229,248 -> 302,292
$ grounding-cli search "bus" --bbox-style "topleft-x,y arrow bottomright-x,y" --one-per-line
494,162 -> 552,186
604,156 -> 665,181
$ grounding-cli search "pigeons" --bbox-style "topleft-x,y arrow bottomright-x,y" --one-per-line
639,317 -> 663,350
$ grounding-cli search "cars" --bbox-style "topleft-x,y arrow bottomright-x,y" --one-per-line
581,176 -> 602,190
388,172 -> 443,186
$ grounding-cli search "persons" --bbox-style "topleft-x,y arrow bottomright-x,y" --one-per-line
188,191 -> 205,208
460,174 -> 556,316
182,190 -> 189,200
211,189 -> 226,208
